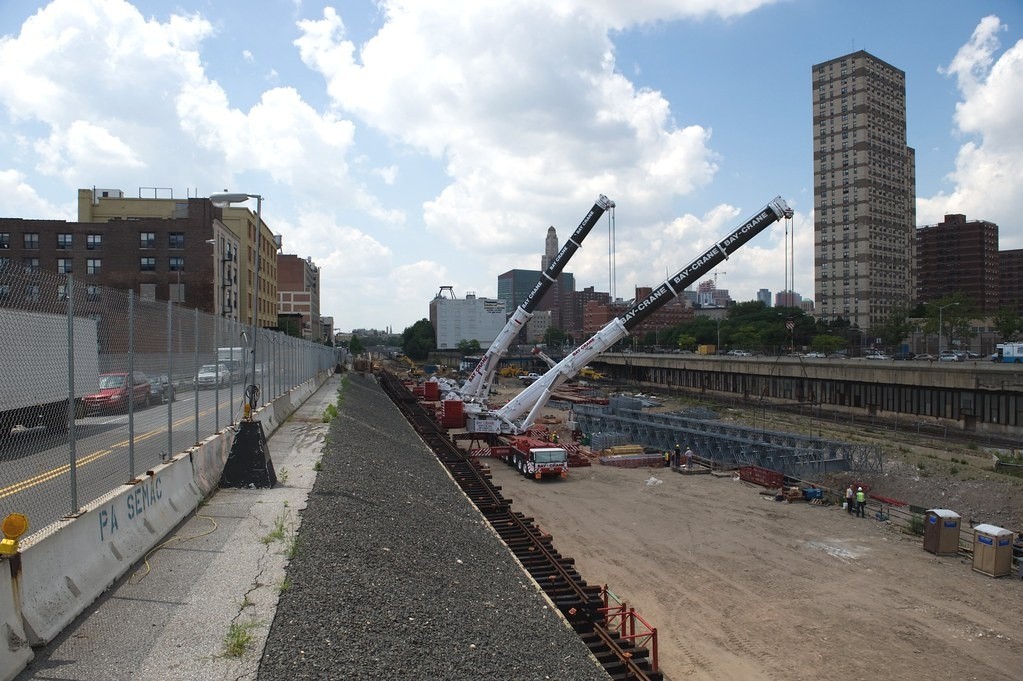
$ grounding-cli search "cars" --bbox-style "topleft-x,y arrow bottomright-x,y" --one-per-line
787,352 -> 895,360
912,350 -> 986,362
519,373 -> 543,380
563,346 -> 577,353
193,364 -> 230,389
255,364 -> 266,377
145,374 -> 176,403
81,370 -> 151,414
624,346 -> 752,357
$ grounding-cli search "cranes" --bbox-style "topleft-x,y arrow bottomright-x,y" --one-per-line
708,269 -> 726,288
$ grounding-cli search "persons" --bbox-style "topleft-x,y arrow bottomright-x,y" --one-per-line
845,484 -> 856,514
856,486 -> 867,518
552,430 -> 559,444
675,444 -> 681,467
665,450 -> 671,467
685,446 -> 693,468
672,451 -> 676,466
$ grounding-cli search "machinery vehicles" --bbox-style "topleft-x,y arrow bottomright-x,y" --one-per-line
398,356 -> 423,377
441,193 -> 615,421
575,366 -> 613,382
501,364 -> 528,377
465,196 -> 794,479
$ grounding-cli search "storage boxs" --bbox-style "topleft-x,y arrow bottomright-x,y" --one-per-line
415,381 -> 438,400
441,400 -> 464,428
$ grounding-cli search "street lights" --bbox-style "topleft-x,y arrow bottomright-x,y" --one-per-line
209,192 -> 261,412
923,301 -> 959,361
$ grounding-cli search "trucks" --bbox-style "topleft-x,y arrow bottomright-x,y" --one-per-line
214,347 -> 252,382
0,306 -> 102,446
992,342 -> 1023,363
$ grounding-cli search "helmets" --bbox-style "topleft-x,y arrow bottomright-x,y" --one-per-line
676,444 -> 679,447
557,436 -> 559,438
553,431 -> 557,433
687,447 -> 689,449
858,487 -> 863,491
